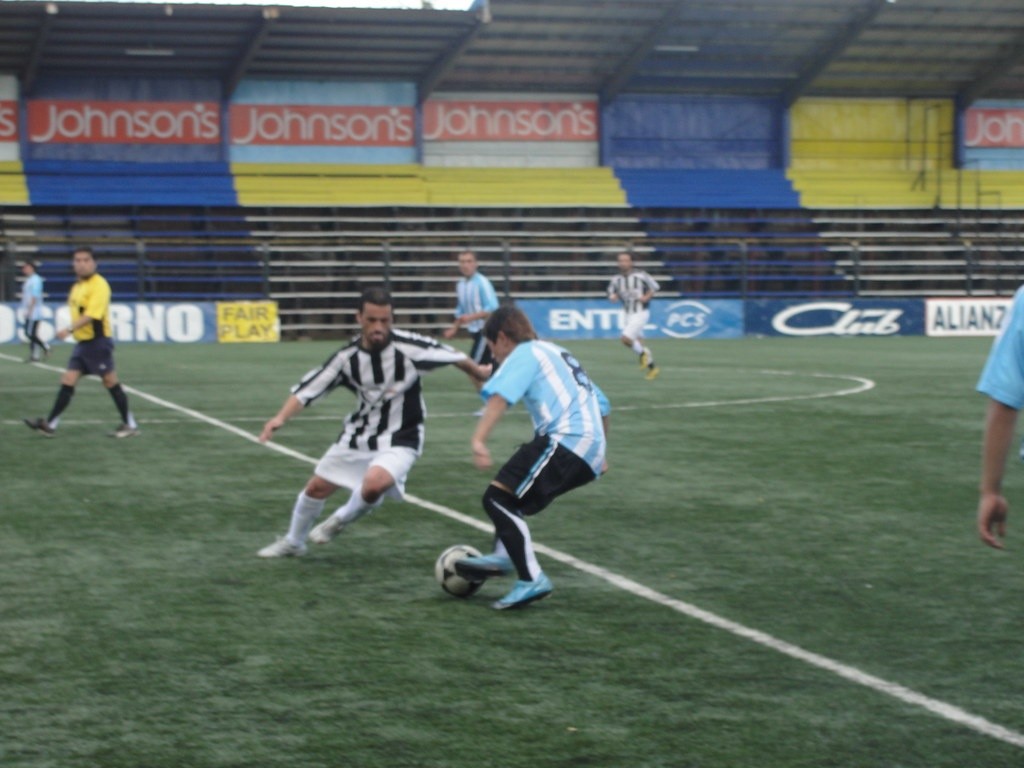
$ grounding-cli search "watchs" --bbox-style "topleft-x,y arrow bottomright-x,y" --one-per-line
66,324 -> 75,334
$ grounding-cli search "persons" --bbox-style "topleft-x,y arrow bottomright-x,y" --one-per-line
22,246 -> 139,440
452,302 -> 615,614
443,251 -> 508,420
256,285 -> 494,559
606,249 -> 661,381
20,259 -> 51,363
974,265 -> 1024,552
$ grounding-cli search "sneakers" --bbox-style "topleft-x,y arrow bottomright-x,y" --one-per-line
646,366 -> 659,379
23,417 -> 55,438
473,407 -> 486,417
257,536 -> 301,558
454,553 -> 515,577
309,509 -> 351,545
640,351 -> 648,369
108,423 -> 142,438
491,572 -> 553,610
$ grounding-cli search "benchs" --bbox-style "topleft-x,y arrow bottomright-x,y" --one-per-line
3,149 -> 1017,331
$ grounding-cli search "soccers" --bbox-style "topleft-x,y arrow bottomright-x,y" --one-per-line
436,544 -> 489,599
